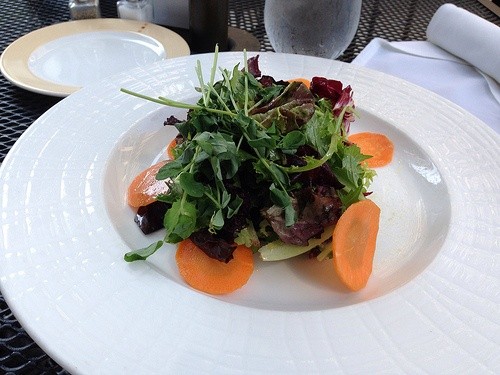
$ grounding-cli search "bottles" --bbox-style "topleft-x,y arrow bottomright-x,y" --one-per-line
69,0 -> 100,20
116,0 -> 154,23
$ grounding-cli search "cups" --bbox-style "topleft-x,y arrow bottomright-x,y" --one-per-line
263,0 -> 362,60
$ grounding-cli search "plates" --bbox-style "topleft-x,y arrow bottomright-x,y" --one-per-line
0,50 -> 500,374
1,17 -> 191,96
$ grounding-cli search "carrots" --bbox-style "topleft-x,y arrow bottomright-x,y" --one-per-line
347,132 -> 393,168
176,235 -> 254,295
332,199 -> 380,291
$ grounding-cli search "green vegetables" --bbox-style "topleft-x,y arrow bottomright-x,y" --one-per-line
119,43 -> 376,262
